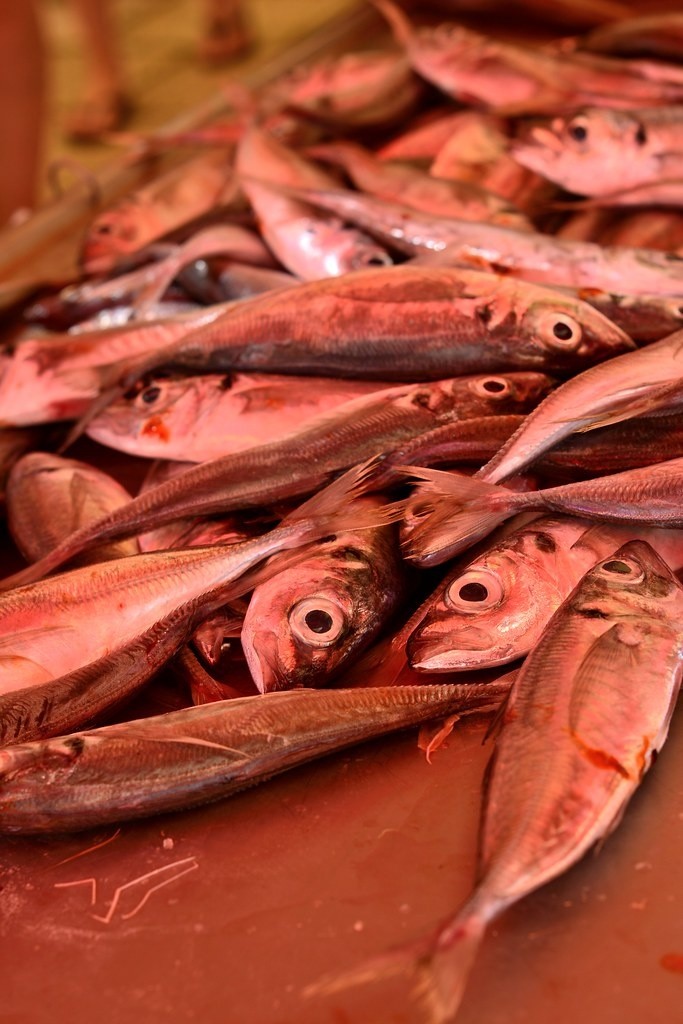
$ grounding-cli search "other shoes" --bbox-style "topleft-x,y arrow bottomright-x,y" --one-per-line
68,95 -> 130,146
202,39 -> 259,69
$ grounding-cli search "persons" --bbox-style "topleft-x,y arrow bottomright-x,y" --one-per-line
0,0 -> 255,219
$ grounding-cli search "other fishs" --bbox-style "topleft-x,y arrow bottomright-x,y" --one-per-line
0,2 -> 683,748
297,539 -> 683,1023
0,684 -> 512,838
